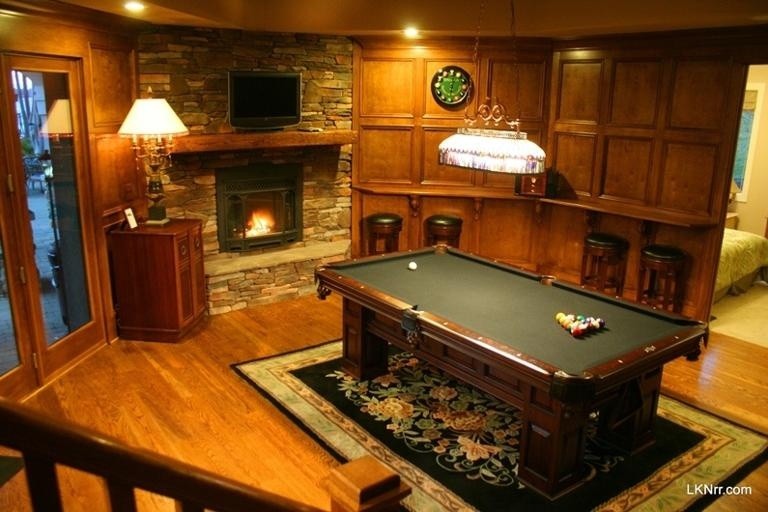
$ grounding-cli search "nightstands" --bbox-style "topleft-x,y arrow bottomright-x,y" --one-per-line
725,212 -> 739,228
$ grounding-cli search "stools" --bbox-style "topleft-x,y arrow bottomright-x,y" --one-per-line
637,245 -> 689,314
427,214 -> 463,248
365,212 -> 402,256
582,231 -> 631,299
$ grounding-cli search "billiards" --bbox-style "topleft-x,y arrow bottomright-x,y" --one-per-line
556,312 -> 604,336
409,262 -> 417,270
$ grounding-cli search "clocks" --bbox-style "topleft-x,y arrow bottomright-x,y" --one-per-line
430,64 -> 473,110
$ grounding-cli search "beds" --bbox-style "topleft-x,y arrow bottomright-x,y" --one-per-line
710,226 -> 768,307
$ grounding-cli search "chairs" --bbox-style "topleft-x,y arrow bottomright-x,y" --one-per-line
23,154 -> 49,194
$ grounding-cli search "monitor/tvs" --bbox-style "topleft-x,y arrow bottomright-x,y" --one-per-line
228,70 -> 302,131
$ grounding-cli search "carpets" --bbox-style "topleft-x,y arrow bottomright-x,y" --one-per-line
0,453 -> 25,491
228,333 -> 768,512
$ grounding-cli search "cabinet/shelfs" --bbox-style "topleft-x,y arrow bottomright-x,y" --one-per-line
108,217 -> 210,343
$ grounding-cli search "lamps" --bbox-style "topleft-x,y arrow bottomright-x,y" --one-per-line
37,99 -> 74,174
728,179 -> 742,211
116,96 -> 191,227
436,0 -> 548,176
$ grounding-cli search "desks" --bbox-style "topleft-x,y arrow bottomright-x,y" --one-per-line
311,245 -> 710,506
